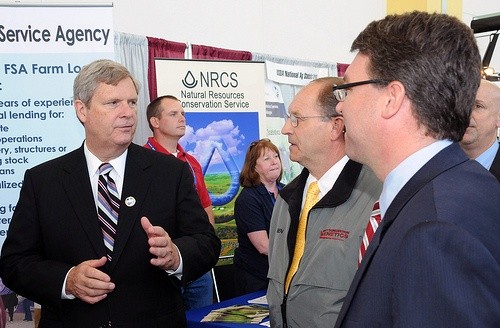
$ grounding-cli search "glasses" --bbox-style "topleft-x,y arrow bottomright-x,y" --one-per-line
333,78 -> 397,102
250,138 -> 270,152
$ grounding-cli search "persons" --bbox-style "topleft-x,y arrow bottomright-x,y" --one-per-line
460,78 -> 500,181
0,59 -> 227,328
266,77 -> 384,327
0,277 -> 34,324
234,138 -> 286,297
143,95 -> 215,309
331,10 -> 500,328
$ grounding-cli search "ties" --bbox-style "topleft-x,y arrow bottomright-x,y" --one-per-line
285,181 -> 320,295
98,162 -> 121,261
357,202 -> 381,269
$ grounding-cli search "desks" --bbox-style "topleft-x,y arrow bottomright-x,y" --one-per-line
185,291 -> 271,328
